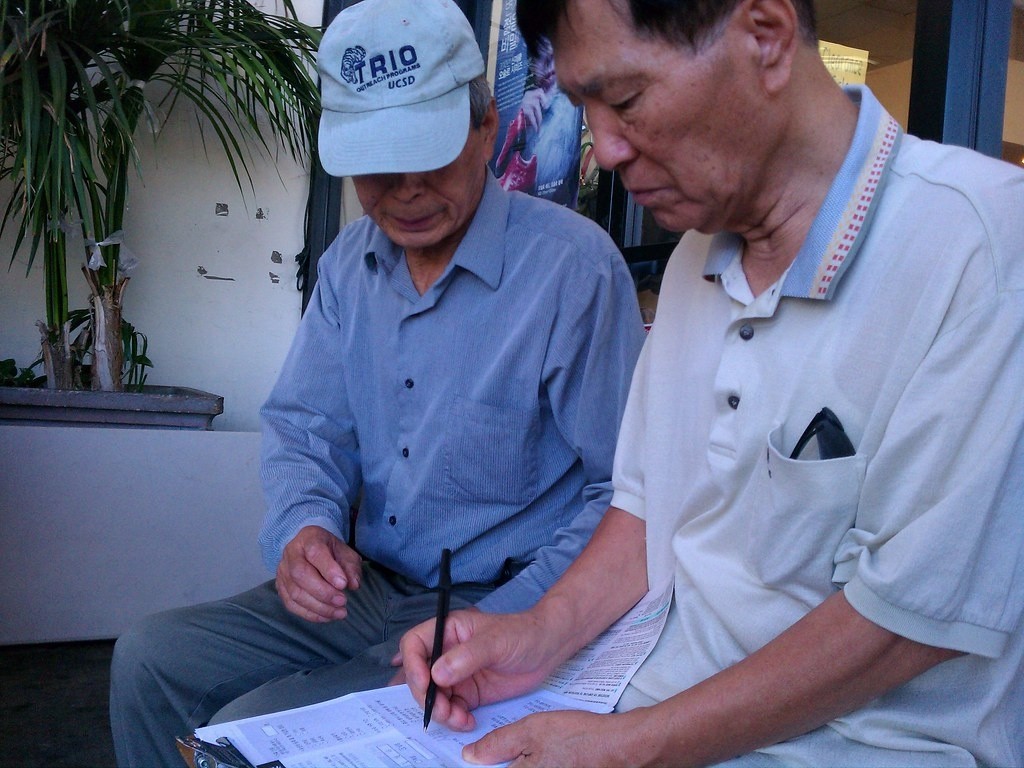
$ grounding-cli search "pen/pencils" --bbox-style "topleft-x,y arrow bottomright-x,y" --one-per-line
406,547 -> 455,731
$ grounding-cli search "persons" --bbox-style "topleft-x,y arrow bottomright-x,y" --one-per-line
496,38 -> 583,207
107,0 -> 645,767
392,0 -> 1024,768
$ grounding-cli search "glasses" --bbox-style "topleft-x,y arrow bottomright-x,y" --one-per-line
791,407 -> 857,461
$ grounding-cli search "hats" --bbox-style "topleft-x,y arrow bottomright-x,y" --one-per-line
316,0 -> 486,177
